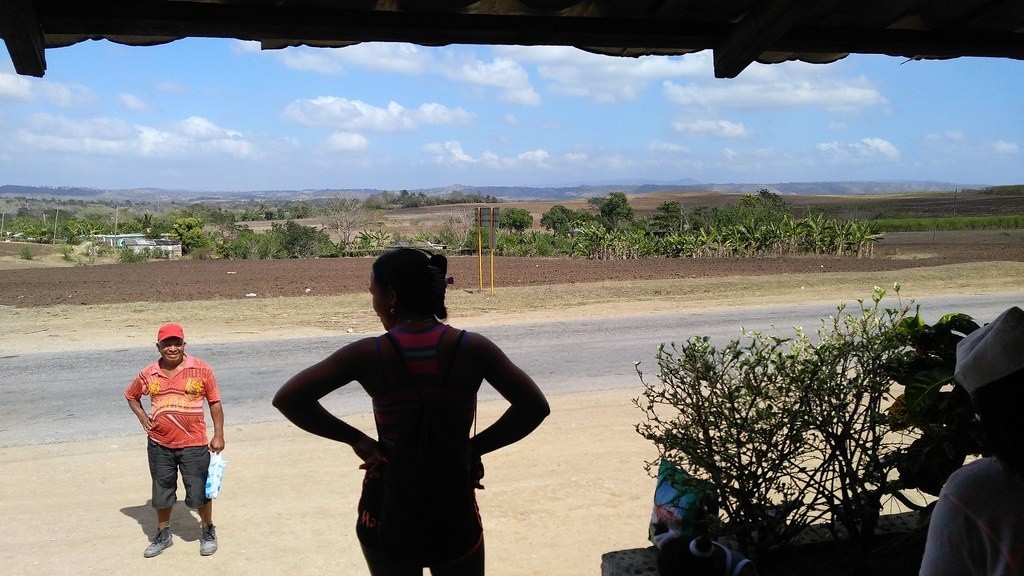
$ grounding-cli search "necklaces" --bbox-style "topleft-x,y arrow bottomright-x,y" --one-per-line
398,318 -> 435,323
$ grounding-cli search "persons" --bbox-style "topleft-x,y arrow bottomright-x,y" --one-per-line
916,307 -> 1024,576
273,246 -> 550,576
124,324 -> 225,558
652,522 -> 757,576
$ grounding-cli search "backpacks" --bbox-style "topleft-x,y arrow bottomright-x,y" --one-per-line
384,330 -> 471,502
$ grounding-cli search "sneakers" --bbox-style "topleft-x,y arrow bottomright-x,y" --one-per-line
200,524 -> 218,556
144,525 -> 173,558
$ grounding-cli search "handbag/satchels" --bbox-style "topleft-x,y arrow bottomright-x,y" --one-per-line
205,451 -> 227,499
648,458 -> 719,550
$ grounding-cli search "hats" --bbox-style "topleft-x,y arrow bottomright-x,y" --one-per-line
953,307 -> 1024,396
158,324 -> 184,342
